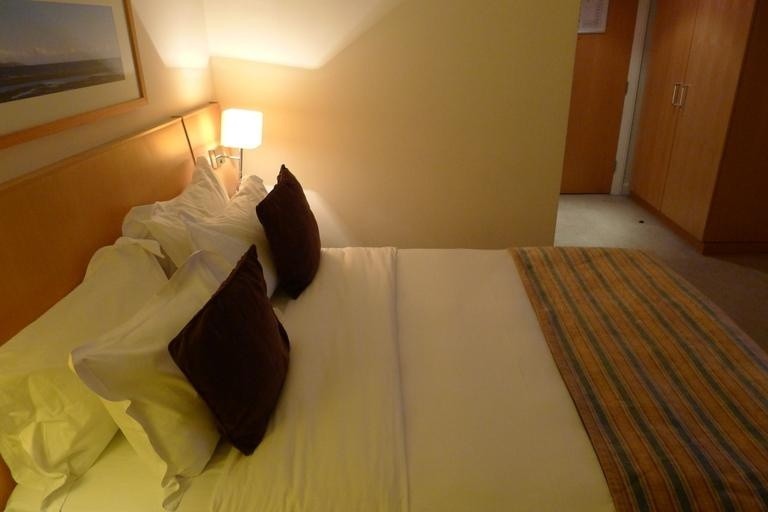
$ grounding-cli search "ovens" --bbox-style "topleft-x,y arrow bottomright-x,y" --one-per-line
1,99 -> 768,512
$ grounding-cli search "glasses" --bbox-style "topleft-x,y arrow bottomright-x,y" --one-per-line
206,106 -> 264,179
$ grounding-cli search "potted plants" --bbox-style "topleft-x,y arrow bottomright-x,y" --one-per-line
579,0 -> 610,35
1,1 -> 153,147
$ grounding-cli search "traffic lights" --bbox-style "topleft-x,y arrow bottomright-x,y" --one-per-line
120,153 -> 233,283
0,237 -> 174,512
66,245 -> 235,512
175,169 -> 281,305
167,243 -> 292,457
256,162 -> 322,300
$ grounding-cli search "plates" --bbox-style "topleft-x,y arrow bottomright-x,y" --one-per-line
628,0 -> 767,254
